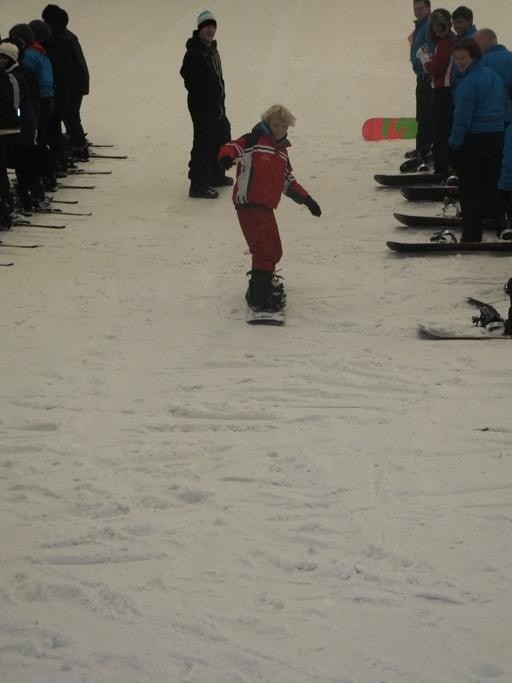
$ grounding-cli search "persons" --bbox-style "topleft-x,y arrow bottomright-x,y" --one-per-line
409,0 -> 512,242
217,105 -> 321,311
0,5 -> 89,231
180,11 -> 233,198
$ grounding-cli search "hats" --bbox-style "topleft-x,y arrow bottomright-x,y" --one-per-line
426,8 -> 452,43
197,11 -> 217,30
0,4 -> 68,63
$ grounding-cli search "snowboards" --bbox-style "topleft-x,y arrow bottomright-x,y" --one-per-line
246,277 -> 284,324
362,117 -> 418,140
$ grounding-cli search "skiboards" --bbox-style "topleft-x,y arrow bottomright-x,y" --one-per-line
374,150 -> 512,340
0,145 -> 128,266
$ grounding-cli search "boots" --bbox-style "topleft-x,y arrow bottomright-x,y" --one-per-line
246,270 -> 287,313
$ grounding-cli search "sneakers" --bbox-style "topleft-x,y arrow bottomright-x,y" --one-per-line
189,176 -> 233,198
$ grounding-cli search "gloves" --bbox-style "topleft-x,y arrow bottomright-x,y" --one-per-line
415,47 -> 431,73
